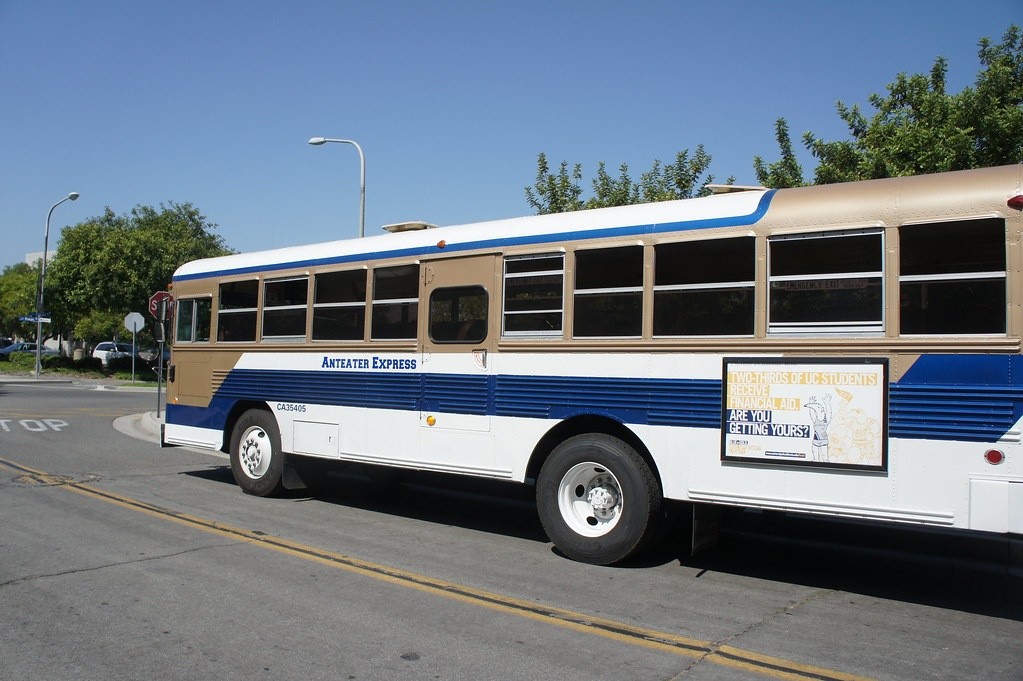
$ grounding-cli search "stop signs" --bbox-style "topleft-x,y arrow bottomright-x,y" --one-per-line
147,290 -> 175,322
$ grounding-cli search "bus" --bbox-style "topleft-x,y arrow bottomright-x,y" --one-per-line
160,157 -> 1023,567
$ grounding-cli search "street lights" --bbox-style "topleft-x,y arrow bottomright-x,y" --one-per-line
33,190 -> 80,377
306,137 -> 368,238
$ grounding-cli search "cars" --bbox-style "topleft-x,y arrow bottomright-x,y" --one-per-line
0,340 -> 61,359
90,340 -> 139,365
